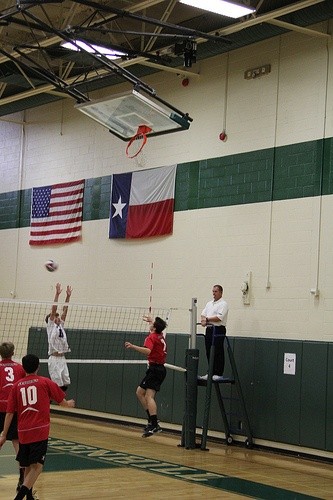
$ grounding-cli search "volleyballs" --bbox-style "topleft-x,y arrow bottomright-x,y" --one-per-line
45,259 -> 58,272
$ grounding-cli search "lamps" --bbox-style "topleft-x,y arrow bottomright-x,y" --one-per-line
179,0 -> 257,20
58,36 -> 129,62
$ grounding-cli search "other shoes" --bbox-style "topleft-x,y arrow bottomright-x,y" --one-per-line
212,375 -> 223,380
200,374 -> 208,380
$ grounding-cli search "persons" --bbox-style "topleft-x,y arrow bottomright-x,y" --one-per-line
201,285 -> 229,381
45,282 -> 73,404
0,354 -> 75,500
125,314 -> 166,439
0,342 -> 26,491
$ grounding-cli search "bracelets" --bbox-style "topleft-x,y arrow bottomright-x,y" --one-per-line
206,317 -> 208,322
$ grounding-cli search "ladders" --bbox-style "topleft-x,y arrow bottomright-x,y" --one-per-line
177,322 -> 254,451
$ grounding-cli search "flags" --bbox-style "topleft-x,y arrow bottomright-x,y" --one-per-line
29,180 -> 85,246
109,164 -> 176,239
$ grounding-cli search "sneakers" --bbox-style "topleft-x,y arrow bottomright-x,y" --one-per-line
141,423 -> 162,438
143,418 -> 159,432
